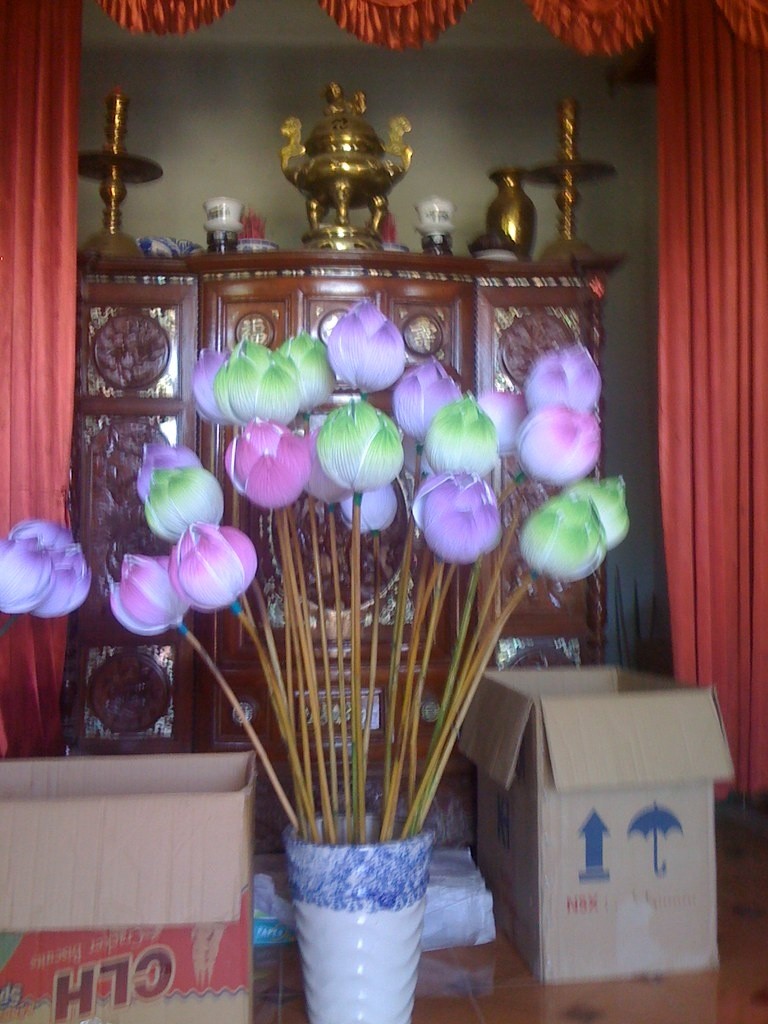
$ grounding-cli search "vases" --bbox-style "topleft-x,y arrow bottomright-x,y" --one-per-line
285,813 -> 434,1024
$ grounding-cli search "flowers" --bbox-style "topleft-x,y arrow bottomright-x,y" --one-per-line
1,301 -> 630,845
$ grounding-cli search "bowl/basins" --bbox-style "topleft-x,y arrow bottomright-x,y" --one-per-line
237,239 -> 280,253
381,244 -> 409,252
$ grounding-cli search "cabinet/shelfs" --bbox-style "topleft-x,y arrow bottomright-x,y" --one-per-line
70,250 -> 628,851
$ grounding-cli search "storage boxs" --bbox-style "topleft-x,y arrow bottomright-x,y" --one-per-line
459,666 -> 736,986
0,751 -> 257,1024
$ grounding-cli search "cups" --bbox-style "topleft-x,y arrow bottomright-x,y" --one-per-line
411,194 -> 456,235
202,196 -> 247,232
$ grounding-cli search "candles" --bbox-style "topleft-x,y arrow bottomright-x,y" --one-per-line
559,95 -> 579,159
106,85 -> 128,150
